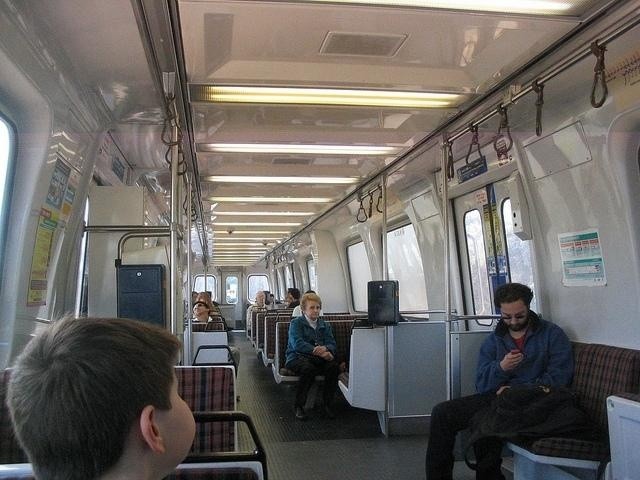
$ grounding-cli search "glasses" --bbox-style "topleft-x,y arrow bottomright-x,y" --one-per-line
192,304 -> 204,312
499,310 -> 525,320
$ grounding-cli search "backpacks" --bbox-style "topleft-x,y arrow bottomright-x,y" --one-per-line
463,382 -> 601,473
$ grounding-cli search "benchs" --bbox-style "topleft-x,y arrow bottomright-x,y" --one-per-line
470,340 -> 640,479
246,301 -> 429,411
0,364 -> 266,480
182,304 -> 237,381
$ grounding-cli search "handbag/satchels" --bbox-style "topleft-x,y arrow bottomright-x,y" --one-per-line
297,339 -> 335,362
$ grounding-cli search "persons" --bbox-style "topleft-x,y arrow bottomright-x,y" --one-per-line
284,294 -> 342,418
280,288 -> 293,308
262,291 -> 275,307
292,289 -> 323,317
425,283 -> 575,479
286,288 -> 300,308
7,316 -> 196,480
191,291 -> 232,330
192,302 -> 240,378
246,291 -> 273,331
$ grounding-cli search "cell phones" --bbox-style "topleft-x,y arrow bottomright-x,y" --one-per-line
510,349 -> 520,355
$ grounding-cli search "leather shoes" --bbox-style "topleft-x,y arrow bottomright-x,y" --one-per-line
295,407 -> 308,419
321,404 -> 336,418
